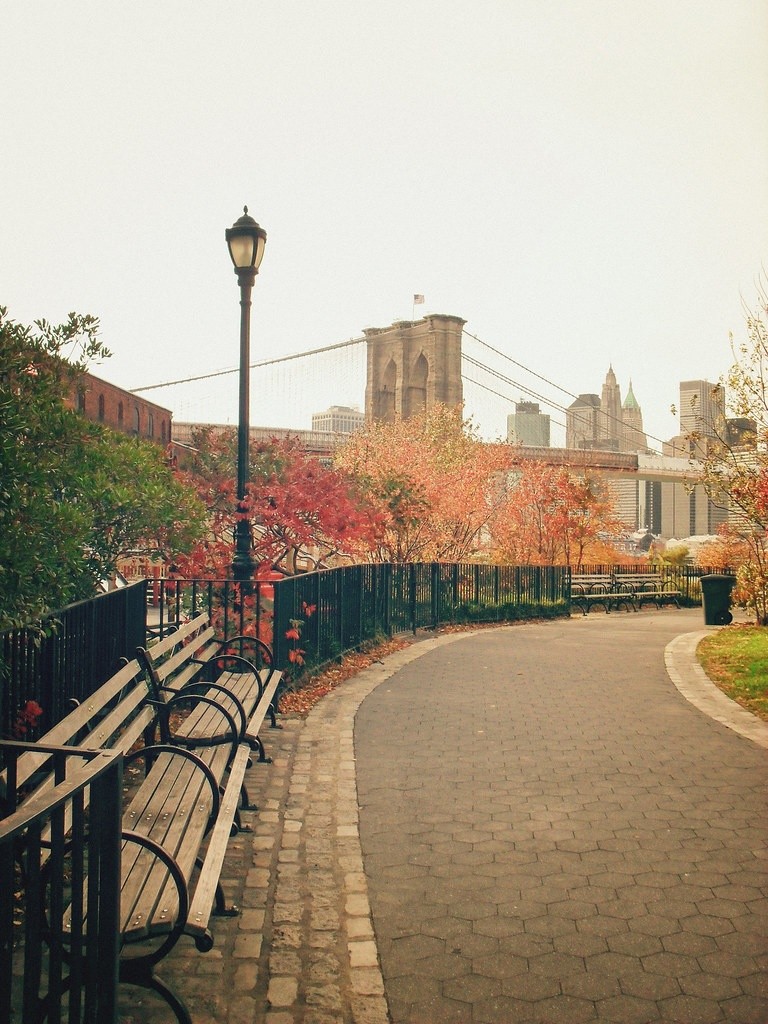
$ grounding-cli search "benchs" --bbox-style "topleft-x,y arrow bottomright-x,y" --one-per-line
561,574 -> 639,617
0,611 -> 287,1024
613,572 -> 682,613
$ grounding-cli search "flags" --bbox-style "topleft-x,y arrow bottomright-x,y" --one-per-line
414,295 -> 424,305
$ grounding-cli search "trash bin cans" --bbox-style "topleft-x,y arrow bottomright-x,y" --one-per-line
701,574 -> 735,626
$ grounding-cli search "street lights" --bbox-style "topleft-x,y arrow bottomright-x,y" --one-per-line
224,204 -> 267,594
